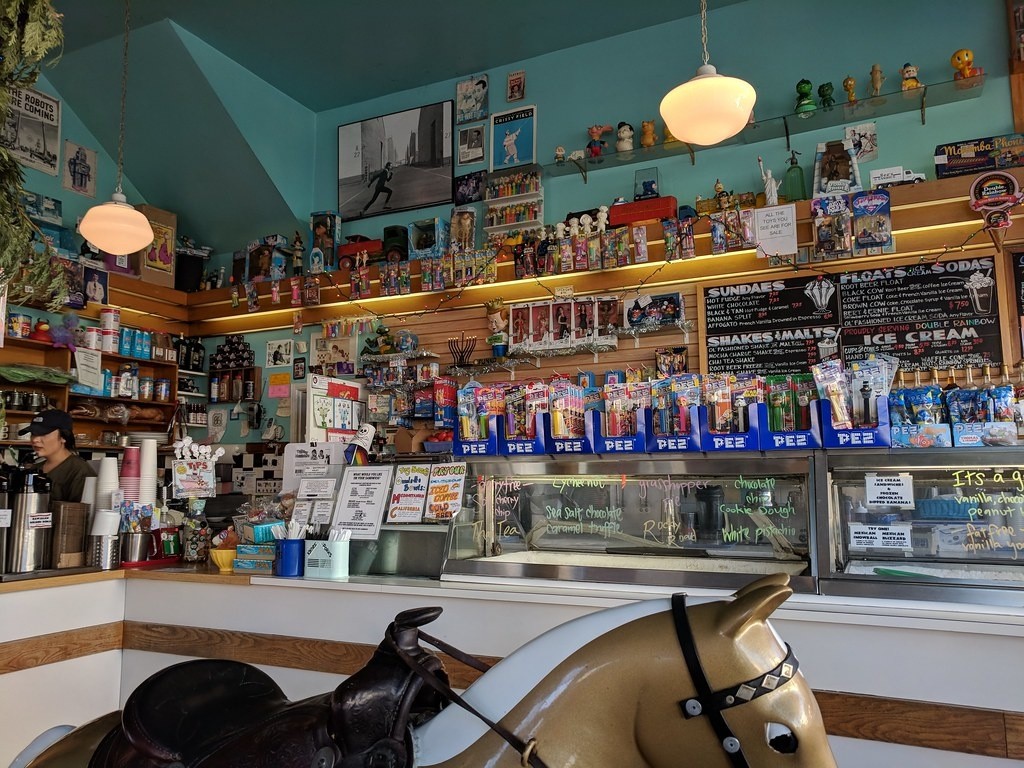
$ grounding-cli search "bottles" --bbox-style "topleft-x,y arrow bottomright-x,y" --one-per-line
188,337 -> 206,373
210,378 -> 219,402
159,506 -> 175,529
219,375 -> 230,402
154,379 -> 171,403
199,265 -> 227,290
233,375 -> 242,402
139,377 -> 153,401
172,332 -> 189,370
897,361 -> 1024,439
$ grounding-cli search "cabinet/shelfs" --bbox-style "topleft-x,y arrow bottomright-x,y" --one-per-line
0,75 -> 1024,613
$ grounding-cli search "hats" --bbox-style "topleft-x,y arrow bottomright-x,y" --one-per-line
18,409 -> 73,436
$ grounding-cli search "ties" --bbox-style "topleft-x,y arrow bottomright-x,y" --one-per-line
94,283 -> 96,288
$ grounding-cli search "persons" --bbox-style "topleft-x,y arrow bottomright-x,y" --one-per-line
459,213 -> 472,249
594,211 -> 609,232
473,80 -> 487,110
15,408 -> 98,503
327,345 -> 353,378
848,130 -> 868,156
758,161 -> 783,205
457,182 -> 482,202
579,214 -> 593,234
290,230 -> 307,277
482,295 -> 508,364
510,303 -> 617,343
358,162 -> 394,216
566,218 -> 581,236
615,121 -> 637,160
86,273 -> 105,304
554,223 -> 566,239
273,345 -> 287,365
824,153 -> 840,182
508,84 -> 521,98
258,248 -> 271,277
470,130 -> 481,147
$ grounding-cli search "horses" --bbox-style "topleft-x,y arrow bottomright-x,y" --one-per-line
8,572 -> 839,768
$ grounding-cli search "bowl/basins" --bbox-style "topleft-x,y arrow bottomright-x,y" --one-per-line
209,548 -> 236,572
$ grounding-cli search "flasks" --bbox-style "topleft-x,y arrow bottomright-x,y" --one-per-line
0,476 -> 13,575
7,473 -> 51,574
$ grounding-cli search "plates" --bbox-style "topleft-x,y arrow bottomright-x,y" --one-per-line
127,431 -> 169,448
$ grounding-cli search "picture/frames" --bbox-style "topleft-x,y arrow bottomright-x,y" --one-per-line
337,100 -> 454,222
82,264 -> 109,307
490,105 -> 536,171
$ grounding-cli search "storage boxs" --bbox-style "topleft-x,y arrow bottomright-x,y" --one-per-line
890,424 -> 953,447
407,216 -> 451,258
953,422 -> 1017,447
130,203 -> 177,288
242,520 -> 285,543
935,133 -> 1024,179
231,558 -> 276,575
308,209 -> 343,272
246,233 -> 288,280
446,205 -> 477,252
236,542 -> 280,560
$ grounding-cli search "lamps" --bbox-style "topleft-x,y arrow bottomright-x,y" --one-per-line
80,0 -> 154,255
659,1 -> 756,146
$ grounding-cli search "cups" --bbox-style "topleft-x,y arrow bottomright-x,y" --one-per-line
660,498 -> 681,541
80,438 -> 158,535
120,531 -> 157,563
272,539 -> 305,577
696,485 -> 725,540
682,512 -> 697,542
348,423 -> 377,455
244,380 -> 254,400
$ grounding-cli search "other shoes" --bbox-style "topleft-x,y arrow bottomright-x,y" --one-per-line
382,205 -> 392,209
359,210 -> 364,215
